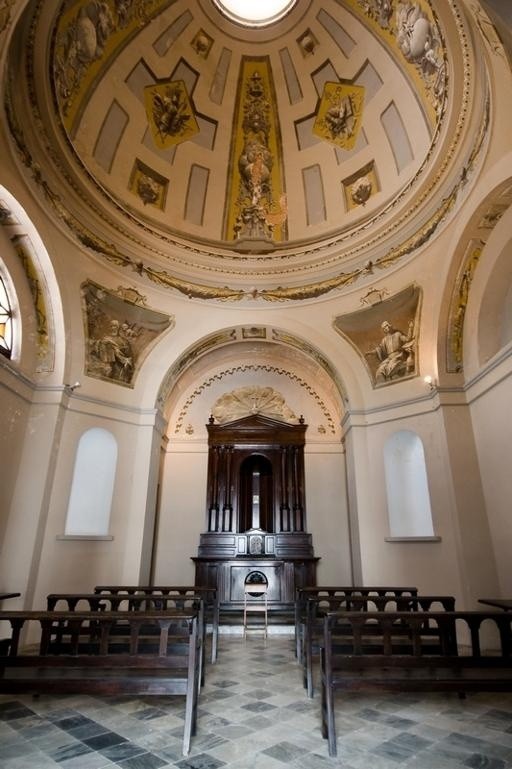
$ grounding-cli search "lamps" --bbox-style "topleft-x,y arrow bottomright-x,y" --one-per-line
72,381 -> 81,390
424,374 -> 433,389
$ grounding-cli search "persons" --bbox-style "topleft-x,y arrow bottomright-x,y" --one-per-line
366,320 -> 413,384
91,319 -> 133,384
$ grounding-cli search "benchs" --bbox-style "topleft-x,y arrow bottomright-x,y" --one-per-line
293,585 -> 510,756
0,584 -> 221,756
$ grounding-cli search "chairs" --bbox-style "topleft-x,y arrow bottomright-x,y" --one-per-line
243,583 -> 267,642
